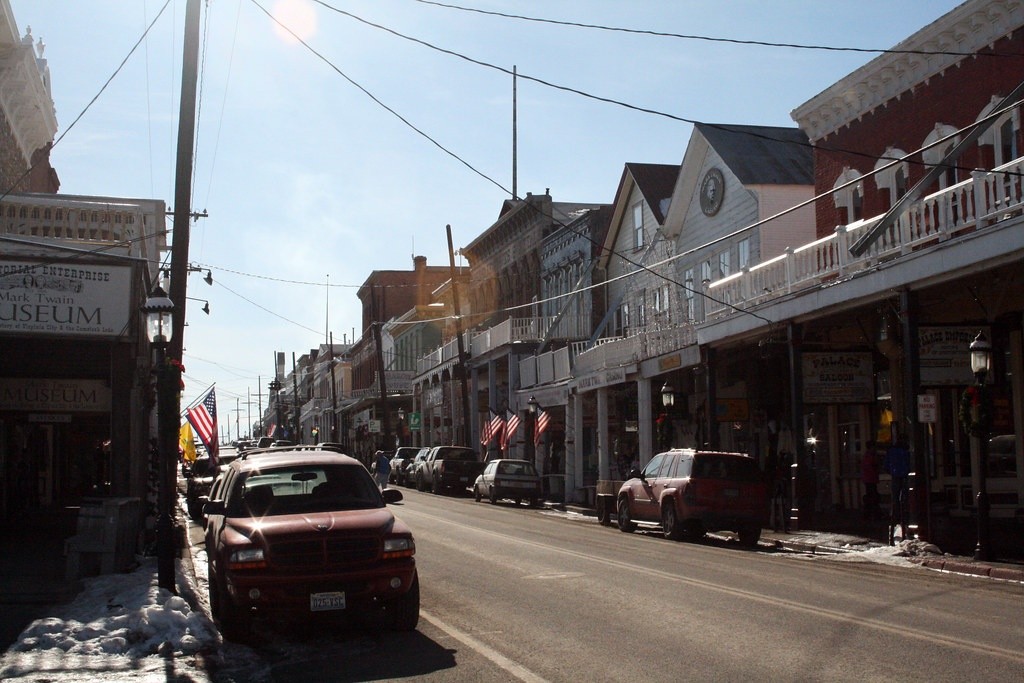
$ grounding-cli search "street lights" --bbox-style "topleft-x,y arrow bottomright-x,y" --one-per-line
968,329 -> 995,560
313,423 -> 321,445
136,281 -> 182,600
398,408 -> 405,448
660,378 -> 675,449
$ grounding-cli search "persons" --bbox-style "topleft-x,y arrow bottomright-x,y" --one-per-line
861,439 -> 880,505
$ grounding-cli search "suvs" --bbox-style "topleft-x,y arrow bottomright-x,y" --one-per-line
616,448 -> 770,547
200,445 -> 419,644
387,447 -> 422,485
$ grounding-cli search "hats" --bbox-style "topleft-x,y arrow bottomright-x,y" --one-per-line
374,450 -> 385,455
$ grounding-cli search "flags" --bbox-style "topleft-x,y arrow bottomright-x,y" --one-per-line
268,423 -> 276,438
179,421 -> 197,461
499,414 -> 522,449
534,402 -> 552,447
186,388 -> 220,468
482,410 -> 503,444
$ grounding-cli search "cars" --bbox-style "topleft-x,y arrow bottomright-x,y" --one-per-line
988,433 -> 1017,472
473,458 -> 540,508
181,437 -> 348,521
404,446 -> 432,488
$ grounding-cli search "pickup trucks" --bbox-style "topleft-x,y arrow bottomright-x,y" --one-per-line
416,445 -> 488,496
